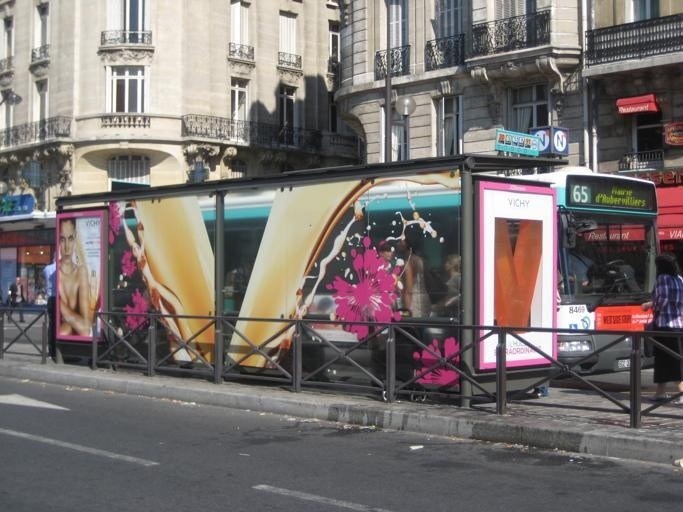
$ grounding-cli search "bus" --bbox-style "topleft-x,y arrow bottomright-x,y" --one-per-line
123,163 -> 661,383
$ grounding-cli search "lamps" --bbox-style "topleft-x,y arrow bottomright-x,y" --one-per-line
395,95 -> 417,120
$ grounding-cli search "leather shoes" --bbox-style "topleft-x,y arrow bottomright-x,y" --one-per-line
674,397 -> 683,405
648,392 -> 667,401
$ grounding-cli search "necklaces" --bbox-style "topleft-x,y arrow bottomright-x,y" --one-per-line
59,266 -> 76,274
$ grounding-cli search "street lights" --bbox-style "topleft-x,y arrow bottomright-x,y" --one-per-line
394,95 -> 419,170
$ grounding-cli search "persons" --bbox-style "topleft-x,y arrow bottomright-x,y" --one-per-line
429,254 -> 461,318
376,236 -> 394,275
7,276 -> 24,324
32,293 -> 47,305
396,227 -> 432,317
639,253 -> 682,400
59,218 -> 93,337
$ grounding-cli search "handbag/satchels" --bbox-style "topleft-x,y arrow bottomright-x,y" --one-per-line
645,322 -> 656,357
